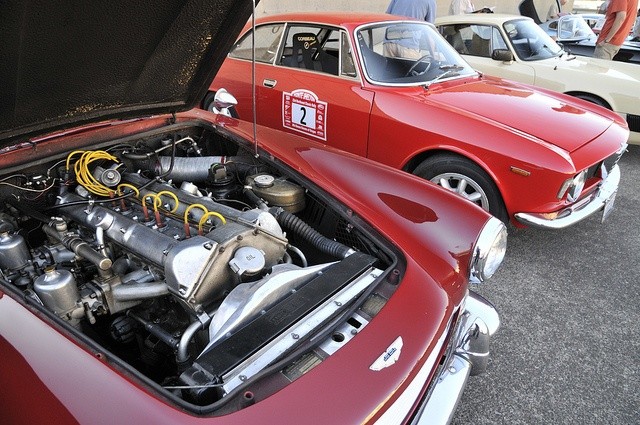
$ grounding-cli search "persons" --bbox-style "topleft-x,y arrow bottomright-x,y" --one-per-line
592,0 -> 639,60
546,0 -> 567,22
383,0 -> 436,59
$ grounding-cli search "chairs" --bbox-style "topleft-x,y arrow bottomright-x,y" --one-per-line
281,30 -> 337,75
447,29 -> 465,54
359,32 -> 389,83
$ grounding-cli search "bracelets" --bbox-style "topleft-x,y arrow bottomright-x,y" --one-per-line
603,39 -> 610,43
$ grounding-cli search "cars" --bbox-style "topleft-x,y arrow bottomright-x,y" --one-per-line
193,6 -> 630,236
509,12 -> 640,65
0,0 -> 510,425
371,11 -> 640,148
